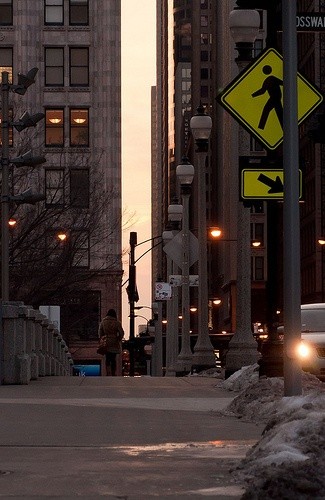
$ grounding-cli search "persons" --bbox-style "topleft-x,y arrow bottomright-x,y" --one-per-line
97,308 -> 124,376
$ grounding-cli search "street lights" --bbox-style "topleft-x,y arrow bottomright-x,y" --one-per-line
225,6 -> 261,378
175,155 -> 195,378
151,196 -> 186,378
189,98 -> 217,377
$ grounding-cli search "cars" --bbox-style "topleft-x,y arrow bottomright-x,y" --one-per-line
265,303 -> 325,380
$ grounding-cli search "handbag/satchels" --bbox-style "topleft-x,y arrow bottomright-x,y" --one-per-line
100,335 -> 113,347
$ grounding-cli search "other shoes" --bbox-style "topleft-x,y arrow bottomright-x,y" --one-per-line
112,374 -> 117,376
106,372 -> 110,376
